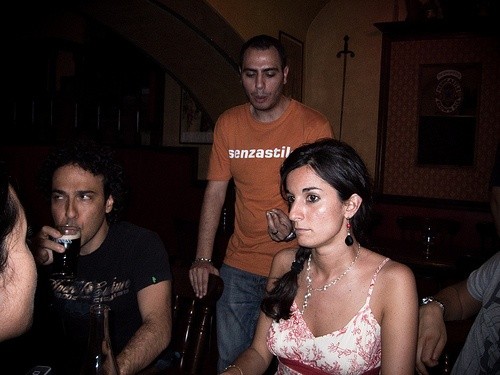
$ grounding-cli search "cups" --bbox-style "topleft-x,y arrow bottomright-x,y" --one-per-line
52,225 -> 81,278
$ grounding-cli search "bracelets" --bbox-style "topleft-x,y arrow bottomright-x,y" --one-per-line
194,258 -> 213,265
223,365 -> 243,375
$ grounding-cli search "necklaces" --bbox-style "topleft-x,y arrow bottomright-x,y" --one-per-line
294,242 -> 361,324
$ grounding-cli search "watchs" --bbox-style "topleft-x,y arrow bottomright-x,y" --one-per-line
420,297 -> 446,312
284,227 -> 296,243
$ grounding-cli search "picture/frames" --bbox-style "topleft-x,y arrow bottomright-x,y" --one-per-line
278,31 -> 304,105
179,81 -> 215,145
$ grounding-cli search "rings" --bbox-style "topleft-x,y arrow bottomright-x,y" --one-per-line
272,230 -> 278,235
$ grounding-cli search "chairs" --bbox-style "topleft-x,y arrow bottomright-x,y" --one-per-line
397,215 -> 462,247
163,273 -> 223,374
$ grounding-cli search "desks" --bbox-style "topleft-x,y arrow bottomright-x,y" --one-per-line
363,239 -> 493,284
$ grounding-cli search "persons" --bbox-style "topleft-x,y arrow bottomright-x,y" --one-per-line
415,251 -> 500,375
221,139 -> 419,375
26,142 -> 173,375
0,174 -> 36,341
189,34 -> 334,375
